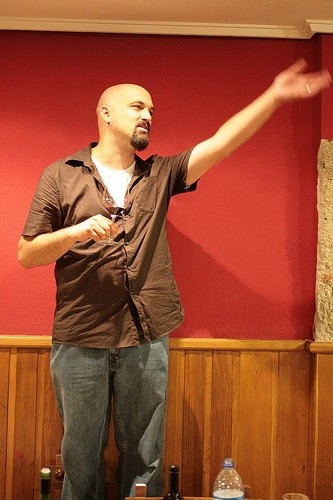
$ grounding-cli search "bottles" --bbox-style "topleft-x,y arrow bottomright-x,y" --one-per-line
213,457 -> 244,500
162,464 -> 185,500
39,468 -> 51,500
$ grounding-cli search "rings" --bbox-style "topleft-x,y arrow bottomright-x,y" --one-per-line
305,83 -> 310,93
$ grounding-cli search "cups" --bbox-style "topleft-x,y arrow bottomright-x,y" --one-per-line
282,493 -> 309,500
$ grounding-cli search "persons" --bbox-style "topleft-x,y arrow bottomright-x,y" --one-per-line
17,57 -> 332,500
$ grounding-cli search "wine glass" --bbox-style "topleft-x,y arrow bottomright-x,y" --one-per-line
98,176 -> 129,246
